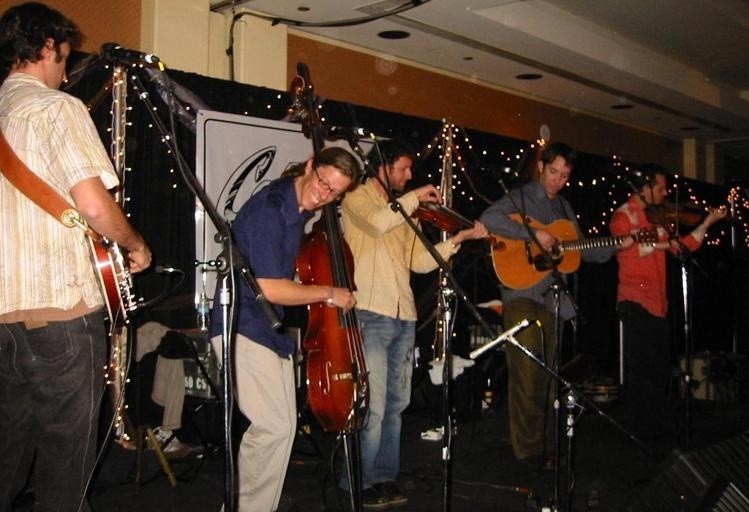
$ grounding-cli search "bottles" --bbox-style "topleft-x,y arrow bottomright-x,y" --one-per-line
195,291 -> 210,332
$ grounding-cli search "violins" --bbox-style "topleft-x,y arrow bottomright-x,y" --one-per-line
643,203 -> 725,225
389,190 -> 497,245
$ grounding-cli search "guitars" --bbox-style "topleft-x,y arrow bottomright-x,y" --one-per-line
489,213 -> 658,290
85,231 -> 137,327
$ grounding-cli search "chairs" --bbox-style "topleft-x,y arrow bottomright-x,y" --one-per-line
133,330 -> 226,491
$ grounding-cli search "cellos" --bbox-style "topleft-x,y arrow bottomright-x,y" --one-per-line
289,63 -> 370,436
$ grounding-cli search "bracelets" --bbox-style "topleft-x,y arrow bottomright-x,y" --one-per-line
667,238 -> 674,249
126,231 -> 146,253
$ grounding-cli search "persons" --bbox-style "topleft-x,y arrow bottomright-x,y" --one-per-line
208,145 -> 361,511
0,2 -> 155,509
336,140 -> 492,509
129,317 -> 198,465
609,163 -> 731,463
478,140 -> 638,474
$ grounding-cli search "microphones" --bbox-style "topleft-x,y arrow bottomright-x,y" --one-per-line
100,40 -> 161,67
319,124 -> 368,140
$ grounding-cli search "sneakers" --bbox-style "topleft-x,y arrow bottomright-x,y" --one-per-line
510,453 -> 560,476
625,425 -> 675,457
338,478 -> 409,509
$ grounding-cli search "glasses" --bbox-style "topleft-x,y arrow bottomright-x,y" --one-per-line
312,161 -> 341,201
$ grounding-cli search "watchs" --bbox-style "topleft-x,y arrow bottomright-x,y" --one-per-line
325,285 -> 335,308
